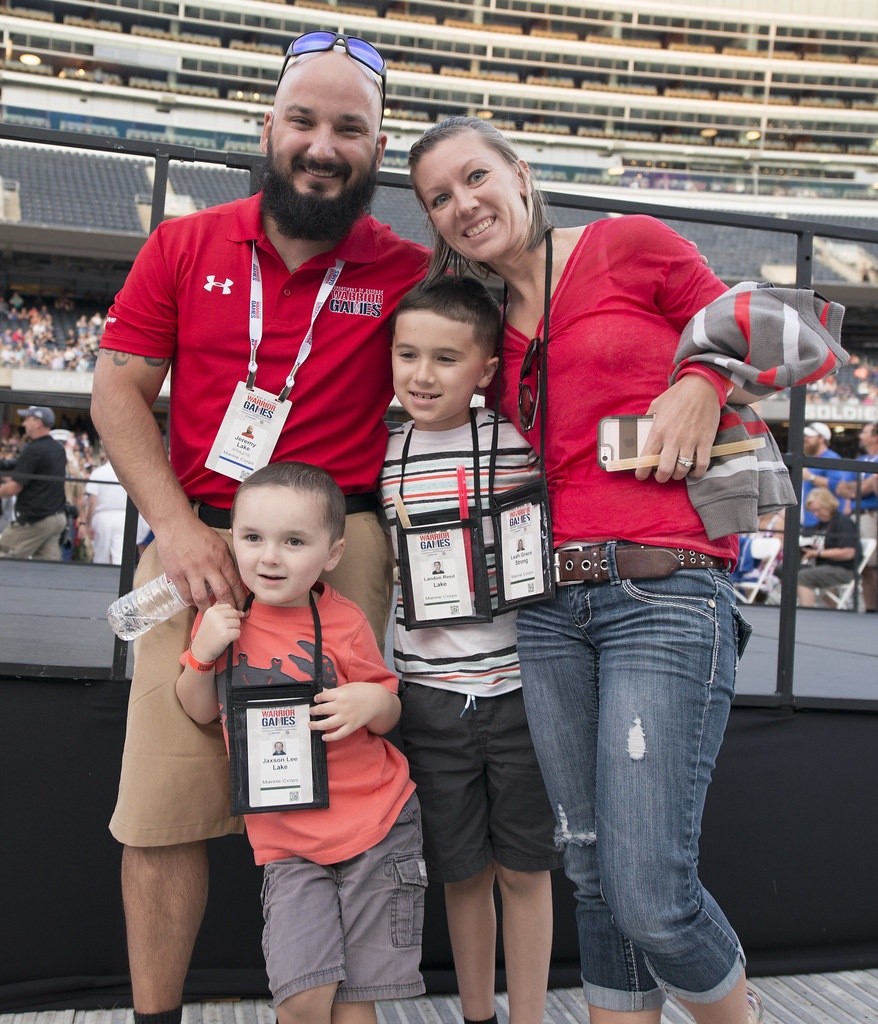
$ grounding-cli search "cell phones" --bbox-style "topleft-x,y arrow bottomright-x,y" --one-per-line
597,415 -> 654,468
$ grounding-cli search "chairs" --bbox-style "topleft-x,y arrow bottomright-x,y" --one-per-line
730,531 -> 878,610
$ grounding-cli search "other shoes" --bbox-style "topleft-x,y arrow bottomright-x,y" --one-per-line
746,987 -> 764,1024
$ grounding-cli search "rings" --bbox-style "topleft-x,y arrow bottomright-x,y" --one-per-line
677,456 -> 694,467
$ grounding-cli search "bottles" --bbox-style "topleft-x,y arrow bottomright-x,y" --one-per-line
107,573 -> 212,641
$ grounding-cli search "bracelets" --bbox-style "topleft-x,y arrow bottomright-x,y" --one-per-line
188,640 -> 214,672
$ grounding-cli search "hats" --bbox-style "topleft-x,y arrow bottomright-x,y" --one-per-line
17,405 -> 56,427
803,422 -> 832,441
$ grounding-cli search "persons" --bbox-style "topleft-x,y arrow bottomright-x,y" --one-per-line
409,117 -> 777,1024
88,34 -> 712,1024
378,278 -> 556,1024
176,461 -> 430,1024
1,290 -> 152,566
432,562 -> 445,575
242,425 -> 255,438
734,353 -> 878,613
517,540 -> 525,552
272,742 -> 285,755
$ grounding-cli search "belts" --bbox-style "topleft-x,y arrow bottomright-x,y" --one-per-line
188,492 -> 378,529
553,538 -> 723,586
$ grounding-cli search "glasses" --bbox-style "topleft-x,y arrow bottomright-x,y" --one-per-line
276,30 -> 386,133
518,335 -> 542,434
